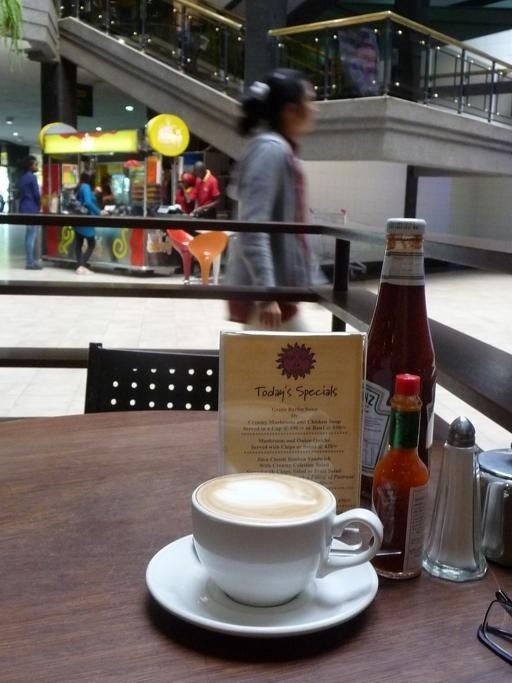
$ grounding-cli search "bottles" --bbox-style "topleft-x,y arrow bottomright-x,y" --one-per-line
362,372 -> 431,583
361,217 -> 436,507
422,416 -> 488,581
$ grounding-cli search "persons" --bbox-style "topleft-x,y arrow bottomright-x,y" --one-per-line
179,159 -> 220,221
18,150 -> 48,271
225,66 -> 323,336
179,173 -> 197,216
64,169 -> 111,276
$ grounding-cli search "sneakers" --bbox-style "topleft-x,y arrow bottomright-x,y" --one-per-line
74,264 -> 94,276
25,263 -> 43,271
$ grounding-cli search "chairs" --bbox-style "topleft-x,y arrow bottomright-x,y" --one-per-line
166,226 -> 239,287
84,341 -> 218,414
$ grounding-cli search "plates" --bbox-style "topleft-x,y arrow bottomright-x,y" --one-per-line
145,533 -> 381,641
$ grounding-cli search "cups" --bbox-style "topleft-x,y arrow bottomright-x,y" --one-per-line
475,439 -> 511,568
191,470 -> 384,607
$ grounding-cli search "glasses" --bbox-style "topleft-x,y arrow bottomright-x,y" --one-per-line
475,588 -> 512,664
